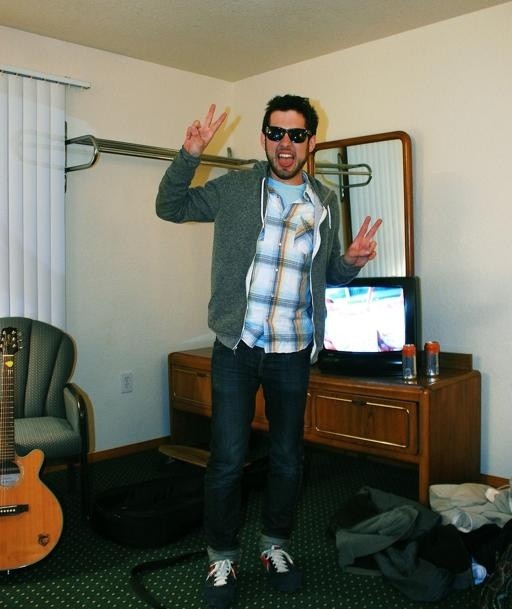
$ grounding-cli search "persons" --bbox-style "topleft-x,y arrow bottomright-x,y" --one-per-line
154,92 -> 386,609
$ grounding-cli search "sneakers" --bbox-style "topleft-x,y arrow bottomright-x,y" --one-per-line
260,545 -> 302,595
202,556 -> 240,607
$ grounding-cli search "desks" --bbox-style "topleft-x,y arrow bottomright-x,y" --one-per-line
166,337 -> 481,510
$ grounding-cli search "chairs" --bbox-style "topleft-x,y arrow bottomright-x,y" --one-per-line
0,315 -> 97,517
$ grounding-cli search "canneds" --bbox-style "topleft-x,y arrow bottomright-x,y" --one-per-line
424,340 -> 440,378
402,344 -> 418,380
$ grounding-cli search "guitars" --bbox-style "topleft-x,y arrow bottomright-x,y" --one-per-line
1,325 -> 64,572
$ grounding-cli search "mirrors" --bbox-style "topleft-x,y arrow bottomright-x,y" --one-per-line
306,130 -> 414,283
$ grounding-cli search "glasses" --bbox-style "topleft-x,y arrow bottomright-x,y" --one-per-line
263,123 -> 312,143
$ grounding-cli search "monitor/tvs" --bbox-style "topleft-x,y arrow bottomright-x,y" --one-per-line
317,277 -> 422,377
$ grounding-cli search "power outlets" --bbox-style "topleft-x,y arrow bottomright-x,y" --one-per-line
120,369 -> 136,394
